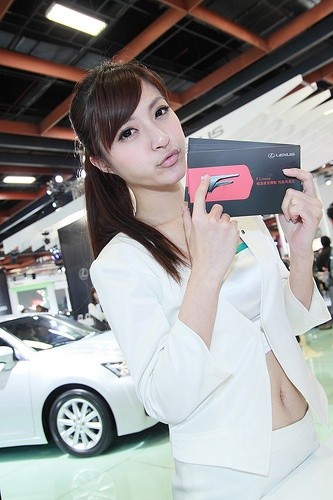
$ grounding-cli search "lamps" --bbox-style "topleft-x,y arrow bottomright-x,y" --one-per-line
44,2 -> 108,37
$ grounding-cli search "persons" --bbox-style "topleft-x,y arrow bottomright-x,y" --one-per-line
69,61 -> 333,500
7,305 -> 70,350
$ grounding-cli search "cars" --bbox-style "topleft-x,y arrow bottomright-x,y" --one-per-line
0,310 -> 159,458
